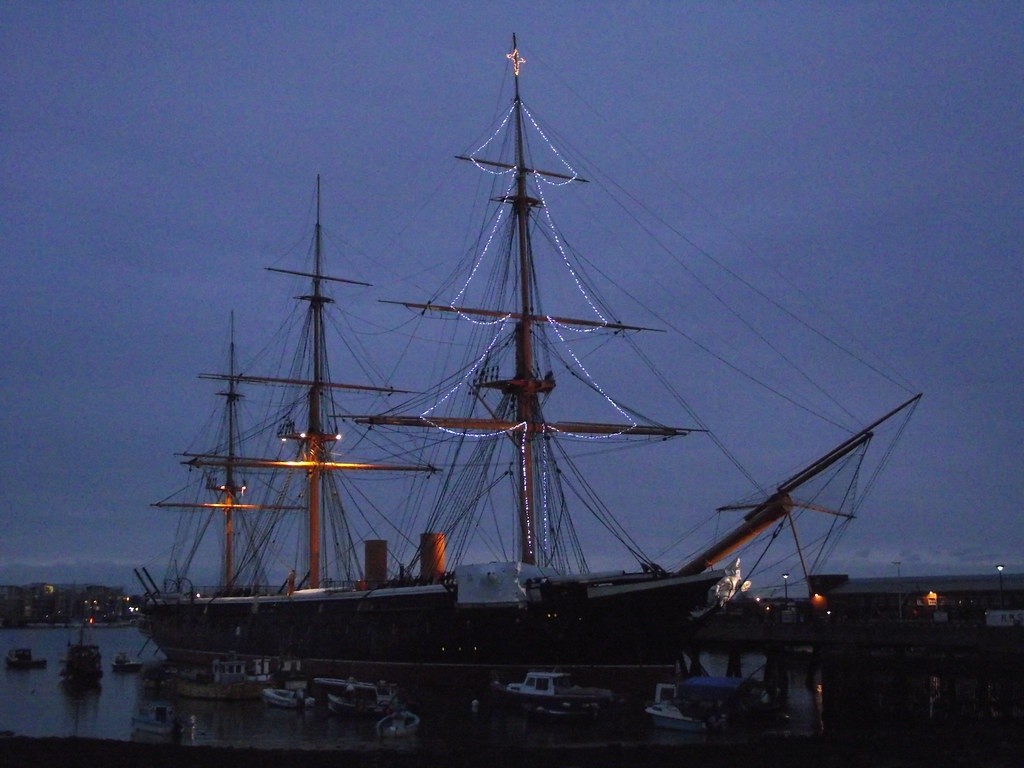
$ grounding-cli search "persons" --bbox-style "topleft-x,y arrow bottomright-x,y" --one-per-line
287,570 -> 297,597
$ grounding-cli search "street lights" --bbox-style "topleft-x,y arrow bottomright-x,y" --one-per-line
996,565 -> 1005,609
782,574 -> 790,592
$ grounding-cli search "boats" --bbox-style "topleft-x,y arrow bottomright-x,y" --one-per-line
487,667 -> 786,735
4,648 -> 47,671
109,641 -> 402,719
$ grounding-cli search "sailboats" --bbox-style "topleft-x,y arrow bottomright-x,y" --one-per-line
59,627 -> 104,698
110,32 -> 923,676
